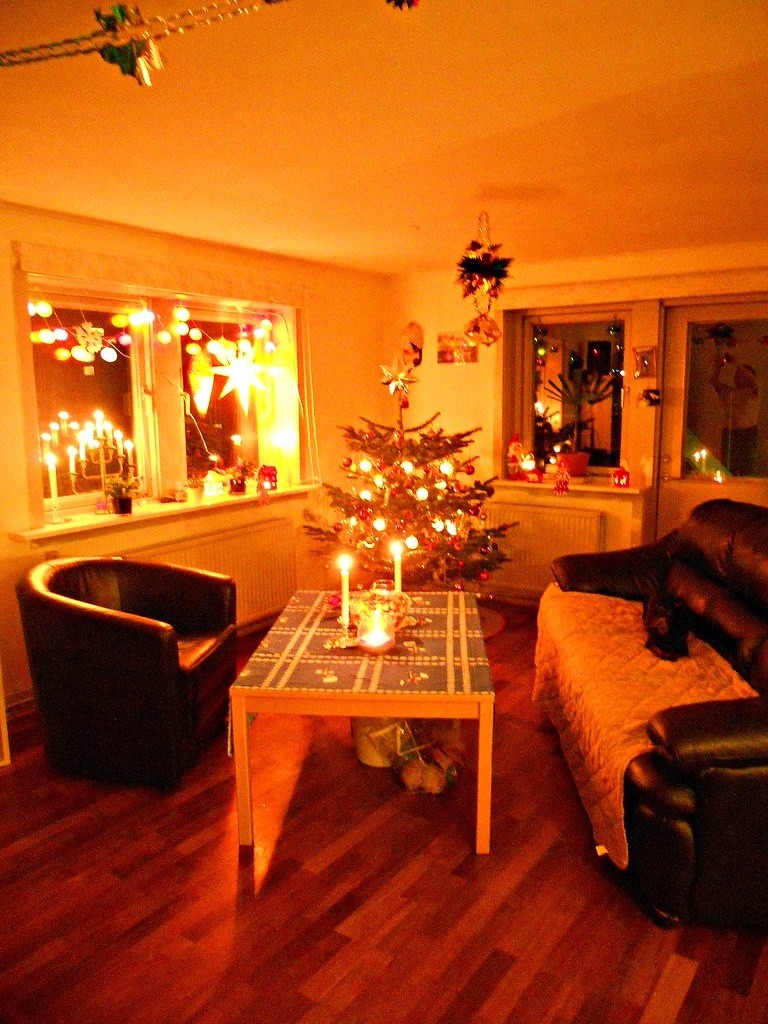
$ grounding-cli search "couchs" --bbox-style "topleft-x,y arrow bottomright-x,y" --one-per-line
531,496 -> 768,932
16,554 -> 239,791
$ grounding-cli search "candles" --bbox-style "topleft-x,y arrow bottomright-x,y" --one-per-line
336,555 -> 355,622
389,541 -> 405,593
40,408 -> 135,473
360,611 -> 395,654
46,454 -> 60,507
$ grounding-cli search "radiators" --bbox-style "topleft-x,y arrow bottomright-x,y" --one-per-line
116,517 -> 296,629
478,502 -> 603,600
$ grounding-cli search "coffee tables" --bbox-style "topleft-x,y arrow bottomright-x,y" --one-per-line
227,588 -> 495,855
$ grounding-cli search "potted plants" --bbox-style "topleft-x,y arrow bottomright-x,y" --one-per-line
187,476 -> 207,501
543,364 -> 621,476
228,466 -> 247,494
102,478 -> 139,516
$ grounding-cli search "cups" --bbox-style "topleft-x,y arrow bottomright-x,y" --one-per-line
357,613 -> 396,654
371,578 -> 395,591
